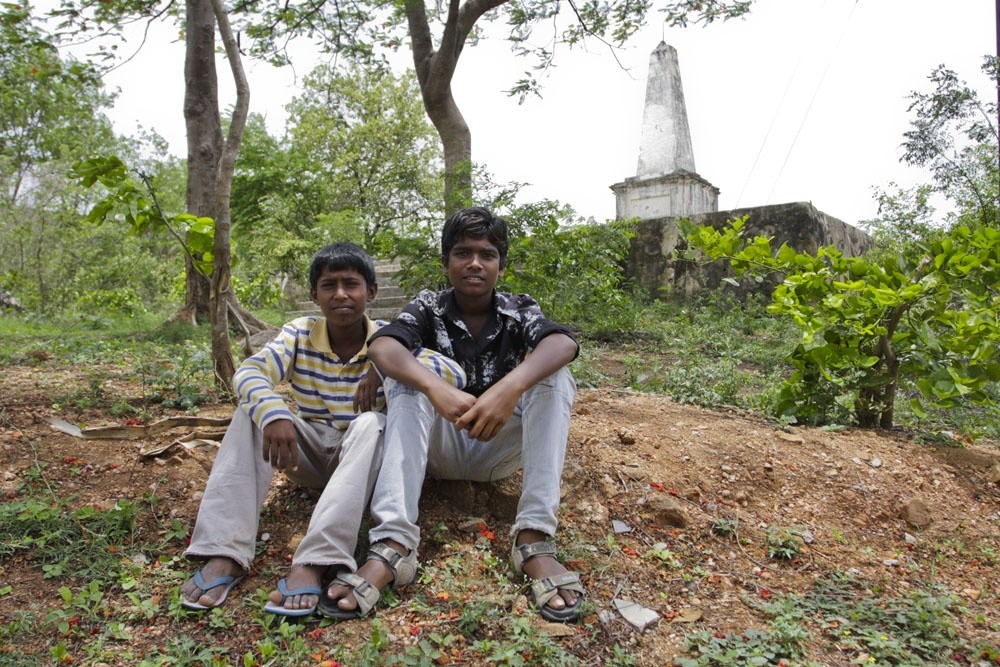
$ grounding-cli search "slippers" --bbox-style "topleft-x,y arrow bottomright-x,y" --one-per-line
180,569 -> 245,609
265,578 -> 324,616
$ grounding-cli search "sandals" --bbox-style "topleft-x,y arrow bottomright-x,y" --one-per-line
507,531 -> 584,620
318,542 -> 417,618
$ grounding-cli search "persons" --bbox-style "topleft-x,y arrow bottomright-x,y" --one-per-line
178,242 -> 467,617
317,208 -> 586,622
